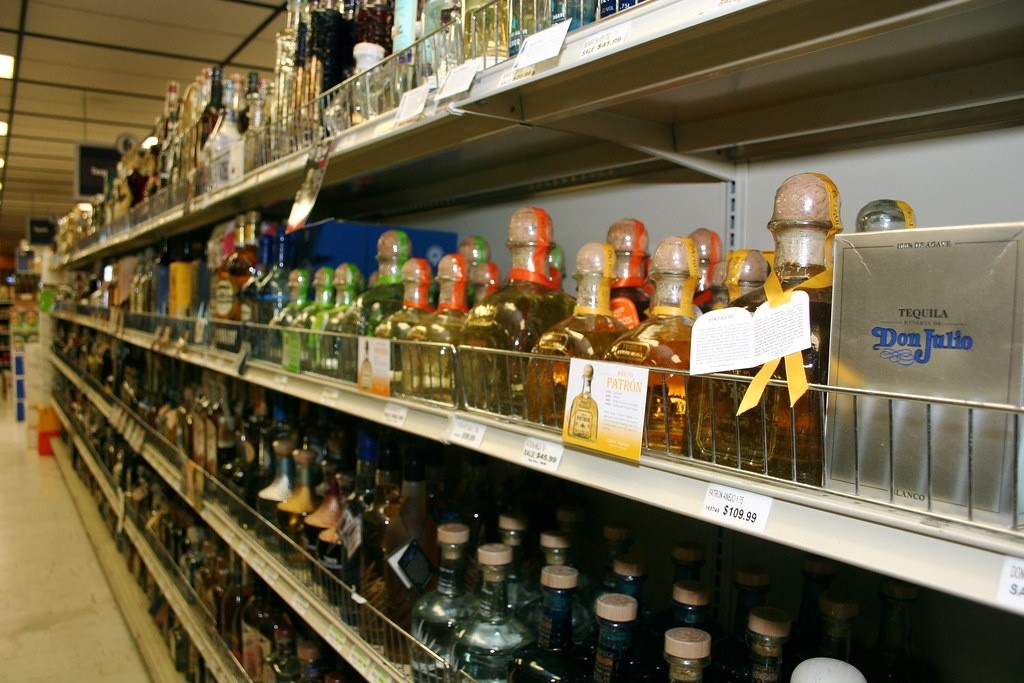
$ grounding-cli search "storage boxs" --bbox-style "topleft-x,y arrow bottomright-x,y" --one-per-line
821,221 -> 1024,528
37,429 -> 61,456
168,261 -> 192,338
258,220 -> 460,292
35,401 -> 62,430
10,345 -> 25,377
149,264 -> 168,333
15,399 -> 25,422
13,378 -> 26,399
192,259 -> 211,315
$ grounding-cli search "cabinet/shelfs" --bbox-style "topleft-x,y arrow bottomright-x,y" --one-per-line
0,0 -> 1024,683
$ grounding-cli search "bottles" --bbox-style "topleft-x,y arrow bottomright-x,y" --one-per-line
46,0 -> 947,683
